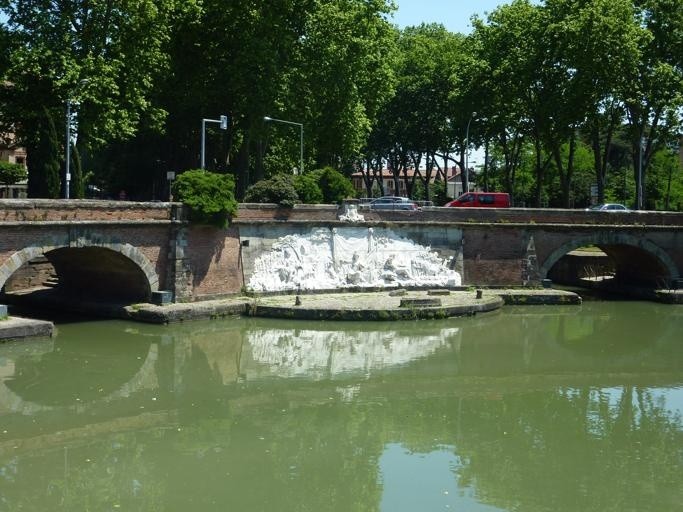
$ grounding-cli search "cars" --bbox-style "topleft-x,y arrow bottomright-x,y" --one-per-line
588,203 -> 628,210
361,196 -> 418,210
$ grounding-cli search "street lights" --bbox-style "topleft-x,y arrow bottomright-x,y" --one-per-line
66,79 -> 90,198
466,111 -> 477,192
638,136 -> 647,209
264,117 -> 304,175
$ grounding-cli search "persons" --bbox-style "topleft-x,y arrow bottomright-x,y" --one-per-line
341,251 -> 369,283
281,253 -> 295,283
385,256 -> 413,280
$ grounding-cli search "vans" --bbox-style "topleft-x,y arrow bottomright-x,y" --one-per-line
442,192 -> 511,207
